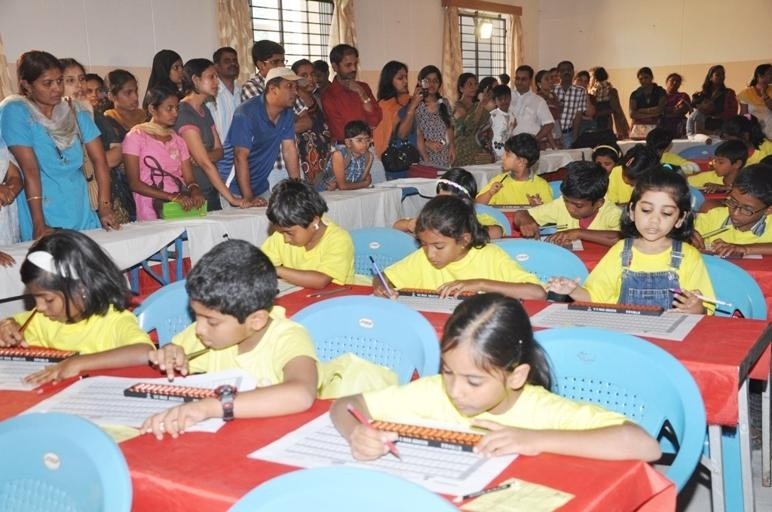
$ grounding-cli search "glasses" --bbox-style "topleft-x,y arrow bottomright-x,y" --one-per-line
423,79 -> 439,84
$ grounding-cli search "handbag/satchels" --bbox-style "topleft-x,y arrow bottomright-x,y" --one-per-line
151,184 -> 208,219
83,157 -> 99,211
408,164 -> 437,178
383,144 -> 419,171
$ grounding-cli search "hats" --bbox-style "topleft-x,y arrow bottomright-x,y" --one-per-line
264,66 -> 305,86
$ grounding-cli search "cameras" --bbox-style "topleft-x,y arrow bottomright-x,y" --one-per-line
417,86 -> 428,97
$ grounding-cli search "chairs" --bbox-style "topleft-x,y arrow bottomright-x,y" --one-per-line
0,411 -> 133,512
128,142 -> 768,512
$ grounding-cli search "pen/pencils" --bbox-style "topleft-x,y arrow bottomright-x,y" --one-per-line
452,484 -> 511,505
540,224 -> 569,228
18,309 -> 36,332
370,256 -> 397,301
669,288 -> 732,307
168,348 -> 209,364
701,227 -> 729,238
499,175 -> 508,182
346,403 -> 400,459
306,287 -> 352,298
